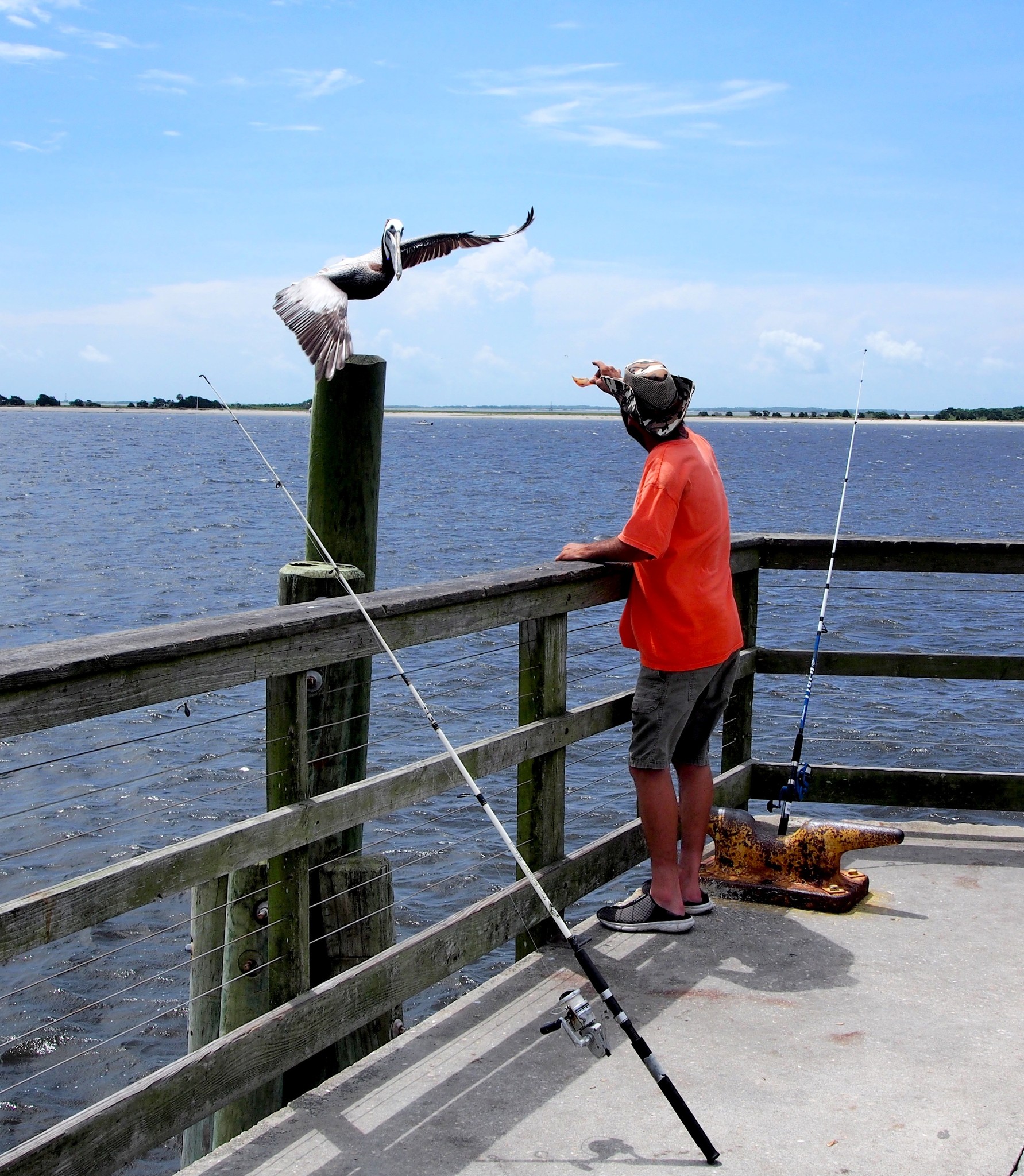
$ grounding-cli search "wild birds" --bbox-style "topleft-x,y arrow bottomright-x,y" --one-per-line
271,205 -> 537,387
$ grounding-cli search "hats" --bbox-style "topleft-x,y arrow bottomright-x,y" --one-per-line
599,359 -> 695,439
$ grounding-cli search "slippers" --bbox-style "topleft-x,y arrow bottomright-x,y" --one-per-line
596,878 -> 714,933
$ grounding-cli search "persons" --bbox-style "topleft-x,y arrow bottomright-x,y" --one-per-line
553,358 -> 745,936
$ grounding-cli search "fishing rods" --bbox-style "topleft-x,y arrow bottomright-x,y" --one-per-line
765,343 -> 880,836
194,373 -> 720,1166
